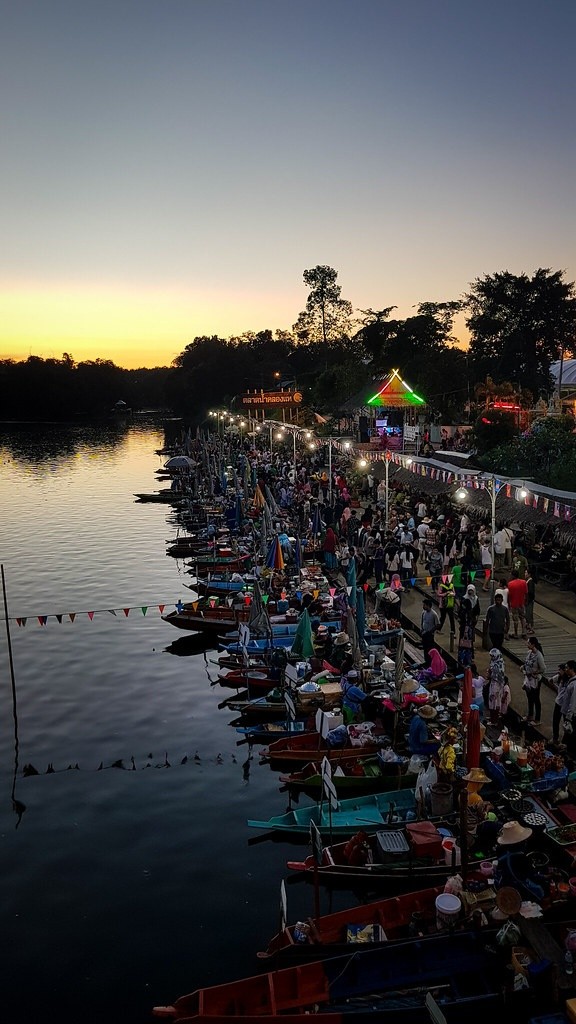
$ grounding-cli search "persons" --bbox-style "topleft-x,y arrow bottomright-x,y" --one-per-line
169,429 -> 576,894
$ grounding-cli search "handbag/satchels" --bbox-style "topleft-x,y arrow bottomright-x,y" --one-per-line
425,561 -> 432,570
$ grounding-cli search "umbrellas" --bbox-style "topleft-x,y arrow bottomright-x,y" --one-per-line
394,633 -> 404,743
347,557 -> 356,611
250,578 -> 271,635
357,587 -> 365,652
264,534 -> 283,590
236,496 -> 245,529
165,457 -> 195,467
253,486 -> 265,509
462,666 -> 474,732
312,505 -> 322,566
468,705 -> 480,771
294,535 -> 302,583
266,487 -> 280,515
291,608 -> 316,667
262,503 -> 273,534
347,607 -> 367,690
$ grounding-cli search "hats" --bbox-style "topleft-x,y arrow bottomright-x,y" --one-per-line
344,670 -> 358,678
497,821 -> 533,845
318,625 -> 327,631
339,538 -> 346,542
373,538 -> 380,544
417,704 -> 438,719
334,632 -> 350,646
400,678 -> 419,693
421,517 -> 433,524
461,767 -> 492,783
350,509 -> 357,514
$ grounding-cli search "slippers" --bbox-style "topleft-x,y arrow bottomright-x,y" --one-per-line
521,716 -> 533,721
529,721 -> 542,726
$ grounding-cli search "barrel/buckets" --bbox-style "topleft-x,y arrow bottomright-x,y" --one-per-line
429,783 -> 453,816
435,893 -> 462,931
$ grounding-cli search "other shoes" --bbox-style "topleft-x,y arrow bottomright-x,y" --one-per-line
511,634 -> 518,639
520,633 -> 528,638
528,629 -> 535,634
525,624 -> 530,629
481,586 -> 489,590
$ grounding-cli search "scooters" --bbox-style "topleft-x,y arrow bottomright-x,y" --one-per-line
414,433 -> 435,458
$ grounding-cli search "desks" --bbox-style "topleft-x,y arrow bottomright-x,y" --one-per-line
388,679 -> 431,699
436,711 -> 485,740
432,731 -> 492,755
497,797 -> 557,829
486,750 -> 568,795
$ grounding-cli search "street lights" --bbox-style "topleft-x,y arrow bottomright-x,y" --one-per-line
240,421 -> 247,448
276,429 -> 314,483
240,418 -> 260,451
307,435 -> 356,506
255,422 -> 285,465
220,411 -> 227,436
228,416 -> 235,441
452,476 -> 538,607
209,411 -> 218,433
358,448 -> 411,531
274,373 -> 281,391
214,411 -> 222,433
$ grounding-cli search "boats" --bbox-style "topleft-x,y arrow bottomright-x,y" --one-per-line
134,430 -> 574,1024
